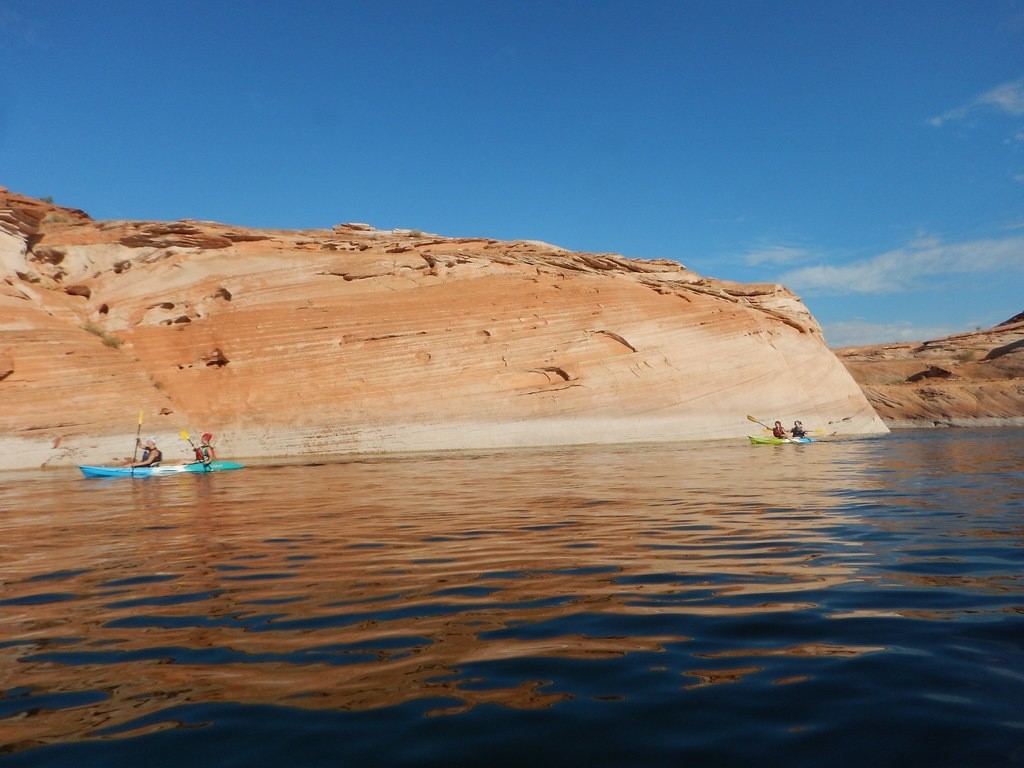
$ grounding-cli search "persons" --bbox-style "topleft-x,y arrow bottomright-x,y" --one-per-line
772,421 -> 788,439
191,433 -> 214,466
787,420 -> 807,439
123,435 -> 162,468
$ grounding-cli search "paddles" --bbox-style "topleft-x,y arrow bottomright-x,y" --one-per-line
179,431 -> 212,471
132,410 -> 145,465
763,429 -> 829,434
748,412 -> 796,445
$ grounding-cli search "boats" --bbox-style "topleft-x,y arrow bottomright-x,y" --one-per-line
76,461 -> 244,478
748,434 -> 820,444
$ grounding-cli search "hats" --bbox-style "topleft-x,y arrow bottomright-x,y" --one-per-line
147,436 -> 157,444
202,433 -> 212,441
